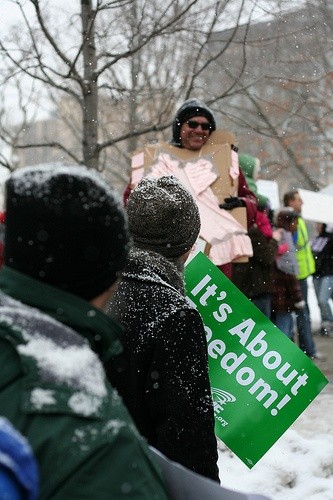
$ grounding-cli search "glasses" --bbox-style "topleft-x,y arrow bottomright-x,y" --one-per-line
186,120 -> 212,130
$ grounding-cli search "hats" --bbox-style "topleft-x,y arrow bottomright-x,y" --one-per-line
171,98 -> 216,143
3,164 -> 129,302
125,175 -> 200,258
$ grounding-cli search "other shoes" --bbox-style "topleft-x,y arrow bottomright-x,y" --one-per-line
312,352 -> 328,361
320,325 -> 333,335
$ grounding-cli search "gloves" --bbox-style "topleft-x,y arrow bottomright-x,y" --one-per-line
272,231 -> 282,242
218,197 -> 246,210
122,183 -> 131,208
294,300 -> 306,308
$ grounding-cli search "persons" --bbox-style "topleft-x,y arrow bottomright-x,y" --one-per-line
103,175 -> 221,485
0,98 -> 333,500
